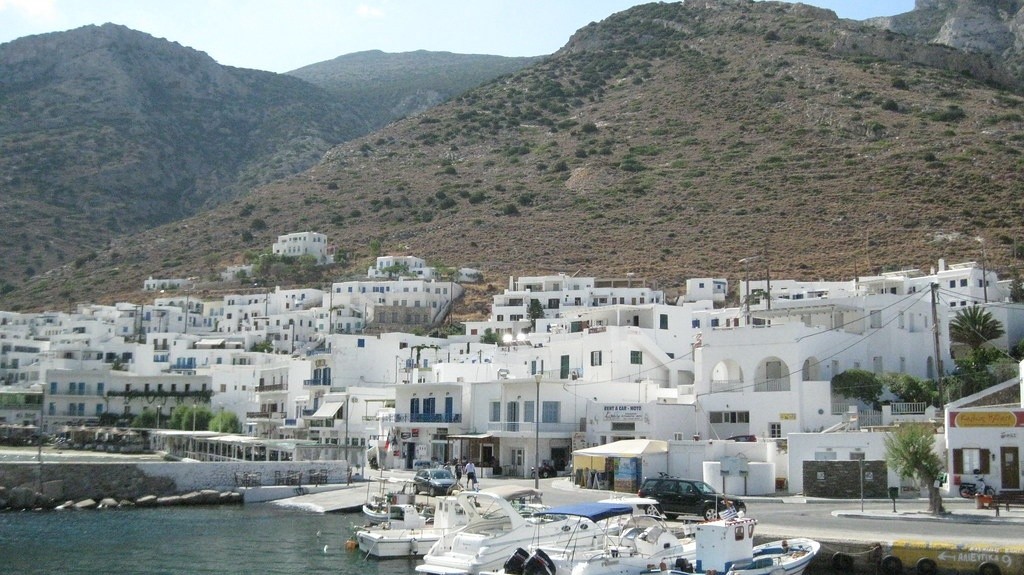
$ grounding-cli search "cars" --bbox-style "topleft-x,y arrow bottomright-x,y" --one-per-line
412,468 -> 459,495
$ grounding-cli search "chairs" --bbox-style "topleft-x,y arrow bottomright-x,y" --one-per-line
234,468 -> 328,486
509,463 -> 517,476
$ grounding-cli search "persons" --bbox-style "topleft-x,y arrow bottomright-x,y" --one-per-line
466,460 -> 475,489
455,460 -> 463,479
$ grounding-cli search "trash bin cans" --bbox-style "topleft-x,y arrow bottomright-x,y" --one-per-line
974,493 -> 993,509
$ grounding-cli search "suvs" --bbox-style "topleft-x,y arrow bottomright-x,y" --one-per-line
637,471 -> 747,520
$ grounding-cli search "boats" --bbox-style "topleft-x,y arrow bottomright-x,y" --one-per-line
358,430 -> 821,575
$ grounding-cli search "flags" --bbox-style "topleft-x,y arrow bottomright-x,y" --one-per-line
383,429 -> 402,456
718,507 -> 739,520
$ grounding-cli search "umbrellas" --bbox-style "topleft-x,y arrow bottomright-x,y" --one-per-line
0,421 -> 140,446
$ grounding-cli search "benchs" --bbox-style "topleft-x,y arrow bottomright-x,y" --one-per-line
990,490 -> 1024,517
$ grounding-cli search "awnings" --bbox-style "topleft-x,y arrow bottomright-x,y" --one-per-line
312,400 -> 344,419
195,338 -> 224,346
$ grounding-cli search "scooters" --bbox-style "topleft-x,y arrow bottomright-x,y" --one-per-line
960,477 -> 996,499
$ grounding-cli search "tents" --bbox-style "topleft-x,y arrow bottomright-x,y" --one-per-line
572,438 -> 667,489
148,428 -> 321,462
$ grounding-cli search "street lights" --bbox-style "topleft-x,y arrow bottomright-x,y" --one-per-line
39,382 -> 45,462
533,374 -> 541,488
346,394 -> 350,461
